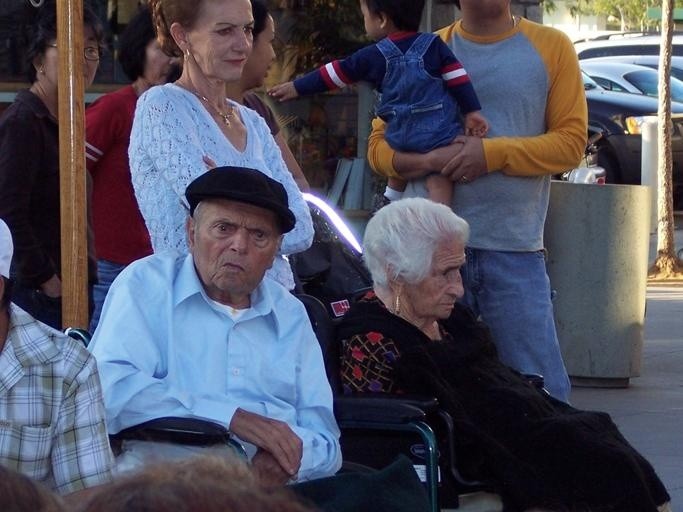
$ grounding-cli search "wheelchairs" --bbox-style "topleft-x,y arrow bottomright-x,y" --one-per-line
272,210 -> 551,510
63,325 -> 444,511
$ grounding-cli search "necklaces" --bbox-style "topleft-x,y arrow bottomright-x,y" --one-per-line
175,80 -> 235,128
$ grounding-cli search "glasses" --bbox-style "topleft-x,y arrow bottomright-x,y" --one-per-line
48,43 -> 105,61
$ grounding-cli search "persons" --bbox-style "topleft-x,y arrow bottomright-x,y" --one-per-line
128,0 -> 314,296
85,14 -> 185,333
225,2 -> 310,195
86,165 -> 342,493
368,0 -> 589,405
335,198 -> 669,512
0,6 -> 108,331
0,217 -> 116,507
266,0 -> 489,210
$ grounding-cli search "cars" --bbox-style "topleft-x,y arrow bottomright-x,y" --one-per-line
557,28 -> 682,82
552,61 -> 683,214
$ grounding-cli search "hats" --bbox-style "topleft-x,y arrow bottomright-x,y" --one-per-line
0,218 -> 13,280
185,166 -> 296,233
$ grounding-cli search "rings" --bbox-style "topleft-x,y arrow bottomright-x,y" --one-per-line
462,176 -> 469,181
